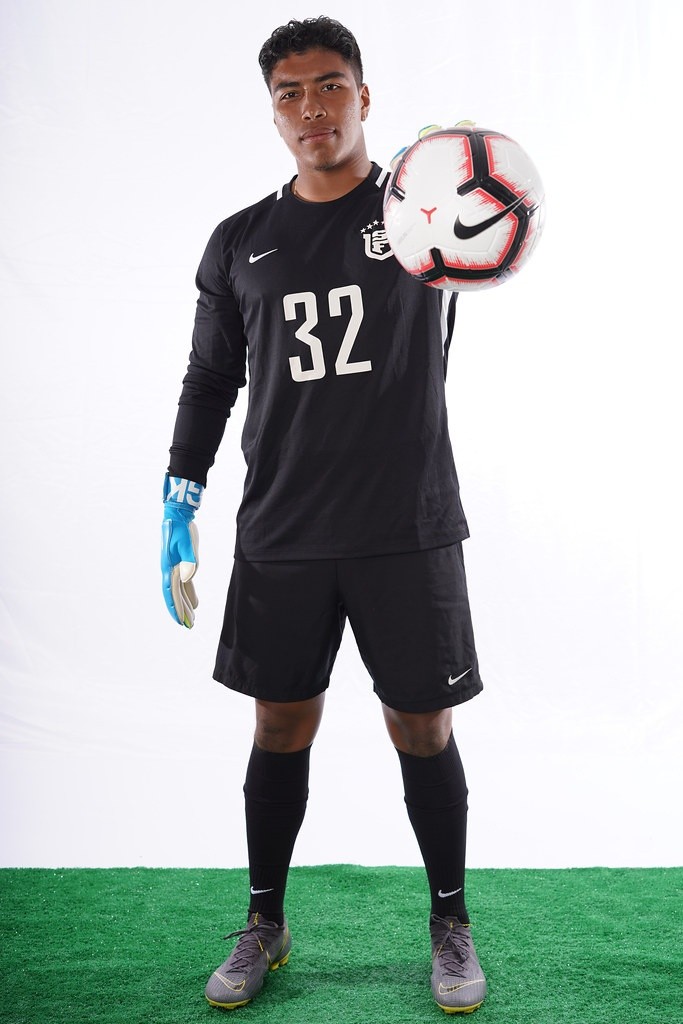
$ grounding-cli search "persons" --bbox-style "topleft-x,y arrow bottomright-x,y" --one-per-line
163,13 -> 486,1014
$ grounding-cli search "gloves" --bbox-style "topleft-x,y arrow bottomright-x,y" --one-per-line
161,472 -> 204,629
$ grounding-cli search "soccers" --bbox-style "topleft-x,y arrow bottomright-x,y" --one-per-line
382,126 -> 543,291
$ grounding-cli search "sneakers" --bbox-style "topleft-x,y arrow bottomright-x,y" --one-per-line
430,914 -> 487,1015
203,909 -> 293,1011
